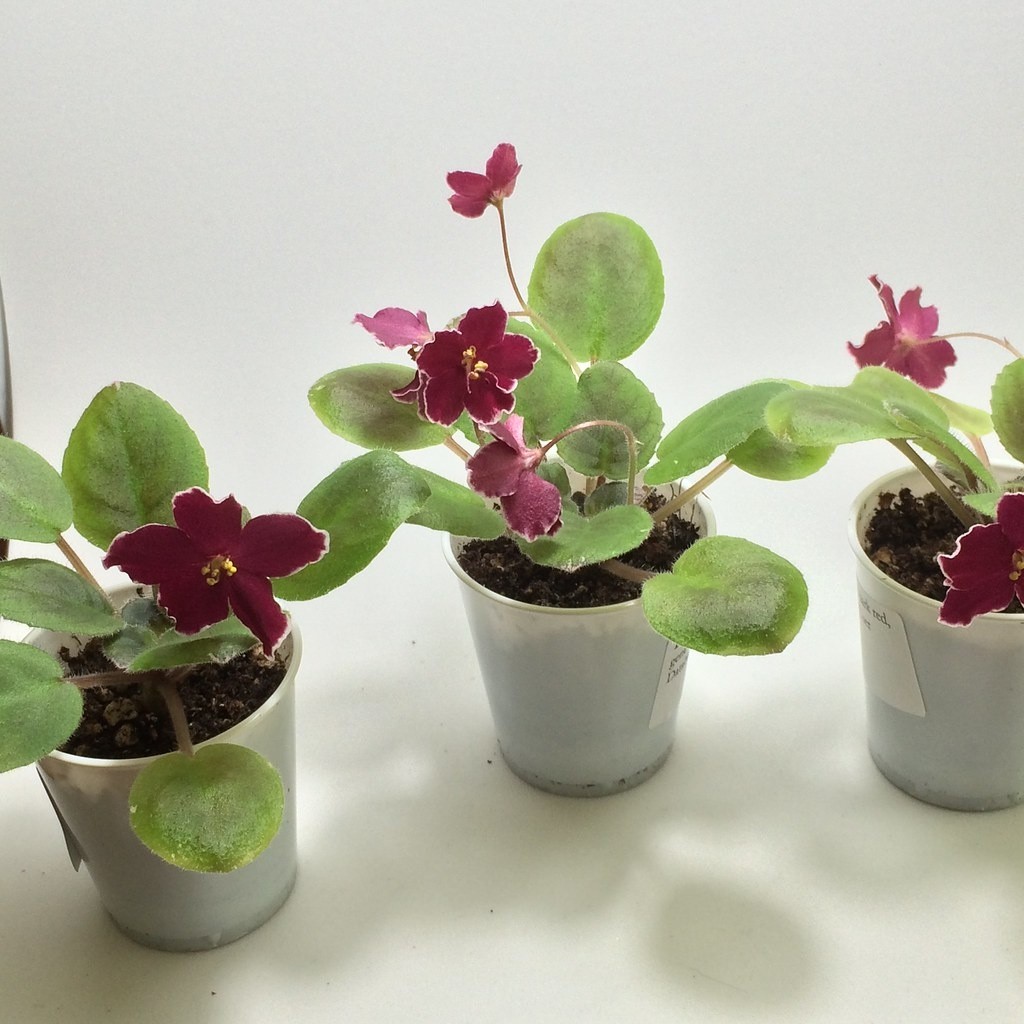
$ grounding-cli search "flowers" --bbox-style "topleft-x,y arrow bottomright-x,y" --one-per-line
0,378 -> 432,874
765,273 -> 1024,627
271,143 -> 838,657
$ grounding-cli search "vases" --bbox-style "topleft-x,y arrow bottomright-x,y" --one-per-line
846,464 -> 1024,814
20,585 -> 303,954
442,452 -> 716,798
